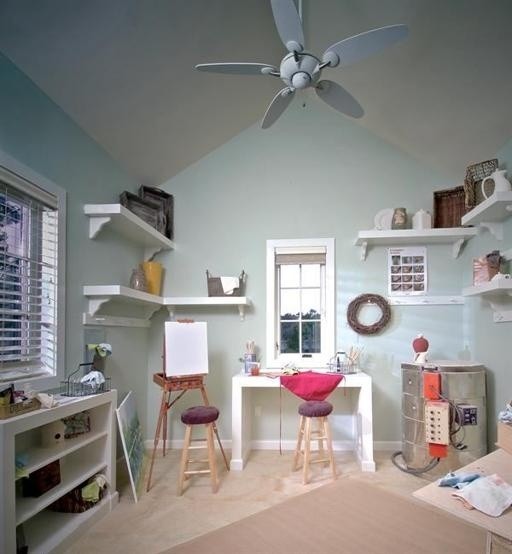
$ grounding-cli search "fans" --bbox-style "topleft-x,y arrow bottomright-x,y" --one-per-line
193,0 -> 407,129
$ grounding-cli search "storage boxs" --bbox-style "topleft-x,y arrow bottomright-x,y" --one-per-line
206,270 -> 245,296
49,482 -> 105,513
494,421 -> 512,457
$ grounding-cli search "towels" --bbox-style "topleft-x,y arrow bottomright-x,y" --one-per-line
450,473 -> 512,518
438,472 -> 481,488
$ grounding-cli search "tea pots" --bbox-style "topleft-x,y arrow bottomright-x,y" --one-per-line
481,169 -> 512,199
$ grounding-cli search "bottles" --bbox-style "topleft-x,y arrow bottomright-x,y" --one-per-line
391,207 -> 407,229
40,420 -> 66,447
129,268 -> 146,291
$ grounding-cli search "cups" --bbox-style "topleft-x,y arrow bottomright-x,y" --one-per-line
141,261 -> 163,296
250,363 -> 260,376
244,352 -> 256,372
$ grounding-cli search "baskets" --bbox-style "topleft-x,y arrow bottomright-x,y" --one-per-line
462,159 -> 498,211
59,363 -> 111,397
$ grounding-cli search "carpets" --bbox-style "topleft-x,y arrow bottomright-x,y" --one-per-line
157,474 -> 512,554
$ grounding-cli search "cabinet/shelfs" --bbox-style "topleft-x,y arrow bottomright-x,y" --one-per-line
0,388 -> 119,553
83,204 -> 252,328
401,362 -> 487,481
352,193 -> 512,323
230,369 -> 375,473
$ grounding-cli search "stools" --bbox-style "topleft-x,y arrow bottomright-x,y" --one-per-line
291,400 -> 338,485
177,406 -> 220,496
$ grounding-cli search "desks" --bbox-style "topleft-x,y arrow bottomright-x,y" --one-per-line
411,449 -> 512,554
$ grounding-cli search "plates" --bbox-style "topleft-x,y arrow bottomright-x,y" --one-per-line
374,209 -> 394,229
